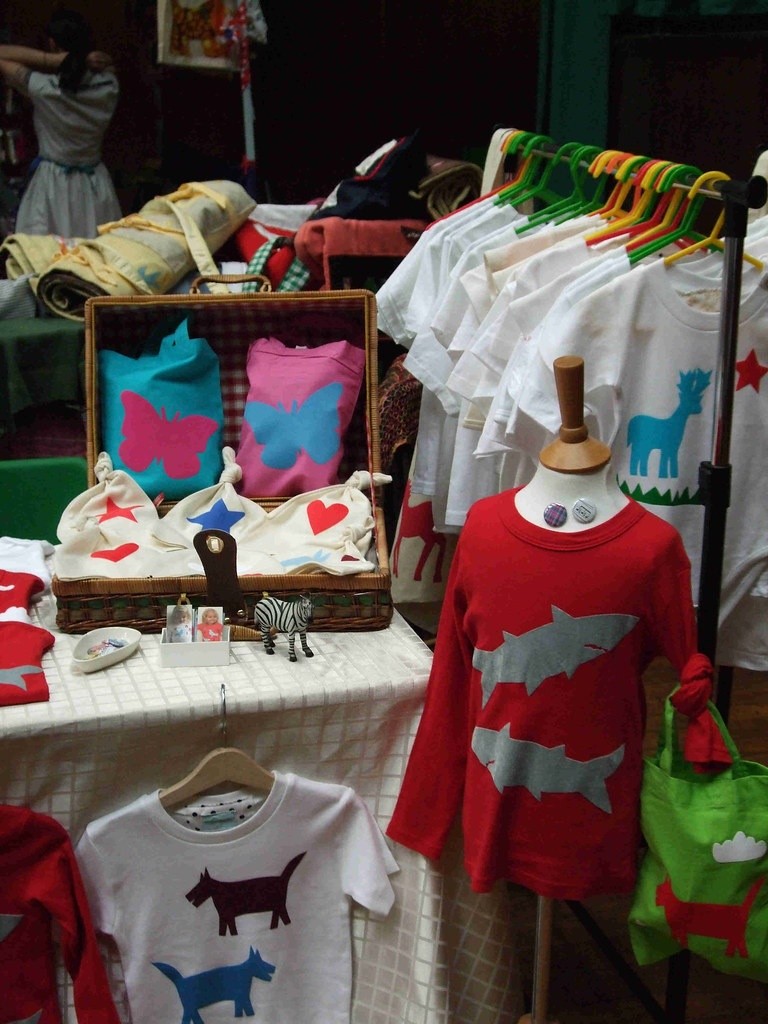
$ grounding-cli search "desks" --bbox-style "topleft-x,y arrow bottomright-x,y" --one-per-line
0,544 -> 535,1024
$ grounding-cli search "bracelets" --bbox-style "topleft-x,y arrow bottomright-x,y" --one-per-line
43,51 -> 46,65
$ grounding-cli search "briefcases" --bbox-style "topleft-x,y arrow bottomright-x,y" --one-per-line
51,275 -> 394,634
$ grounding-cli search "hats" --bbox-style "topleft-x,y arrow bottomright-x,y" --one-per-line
54,446 -> 393,582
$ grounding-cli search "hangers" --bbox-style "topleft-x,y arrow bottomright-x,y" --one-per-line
157,684 -> 275,810
497,131 -> 765,274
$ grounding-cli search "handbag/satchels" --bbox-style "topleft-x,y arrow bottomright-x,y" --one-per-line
626,684 -> 768,984
236,326 -> 366,499
99,309 -> 226,502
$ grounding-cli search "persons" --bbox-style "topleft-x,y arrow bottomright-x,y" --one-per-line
385,353 -> 735,1024
0,11 -> 124,239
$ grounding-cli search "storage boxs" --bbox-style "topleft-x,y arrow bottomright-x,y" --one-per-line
161,627 -> 231,667
